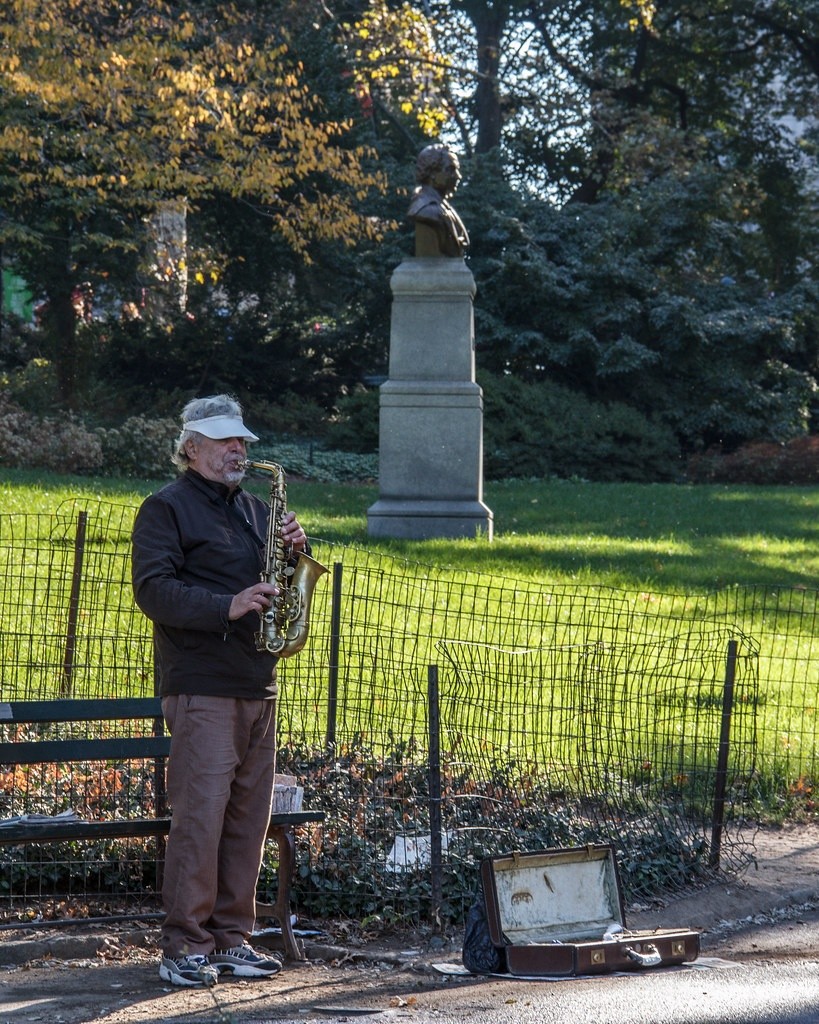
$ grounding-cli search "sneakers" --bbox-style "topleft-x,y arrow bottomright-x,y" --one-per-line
158,954 -> 218,986
207,942 -> 281,976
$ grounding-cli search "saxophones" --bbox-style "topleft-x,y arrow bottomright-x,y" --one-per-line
232,455 -> 336,662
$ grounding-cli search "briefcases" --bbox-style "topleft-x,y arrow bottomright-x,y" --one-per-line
479,843 -> 701,977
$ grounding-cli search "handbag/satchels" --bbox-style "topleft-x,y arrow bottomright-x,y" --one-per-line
462,882 -> 503,974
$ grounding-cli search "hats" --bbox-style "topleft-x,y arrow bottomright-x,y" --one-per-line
182,415 -> 260,443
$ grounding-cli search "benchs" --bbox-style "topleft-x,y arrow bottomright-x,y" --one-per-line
0,698 -> 327,958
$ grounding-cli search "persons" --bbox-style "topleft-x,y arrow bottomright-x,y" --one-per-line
408,143 -> 470,257
131,394 -> 313,987
